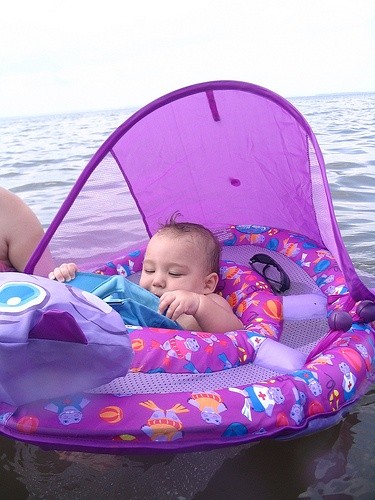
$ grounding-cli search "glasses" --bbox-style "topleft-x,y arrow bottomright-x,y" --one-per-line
249,254 -> 290,296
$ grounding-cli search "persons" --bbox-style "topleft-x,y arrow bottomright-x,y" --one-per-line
0,186 -> 56,279
49,209 -> 243,334
